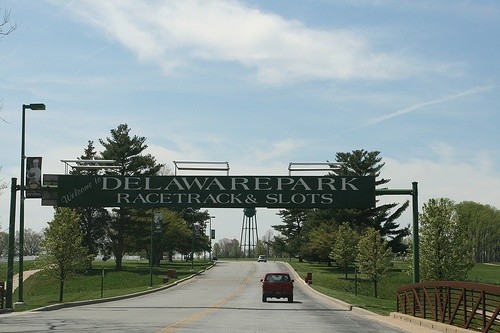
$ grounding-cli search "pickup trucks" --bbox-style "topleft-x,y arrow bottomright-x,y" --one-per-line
184,255 -> 194,259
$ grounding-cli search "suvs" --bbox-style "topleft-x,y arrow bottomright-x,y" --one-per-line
260,273 -> 294,302
258,255 -> 266,262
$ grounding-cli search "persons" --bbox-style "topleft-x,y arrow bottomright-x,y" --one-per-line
279,275 -> 287,281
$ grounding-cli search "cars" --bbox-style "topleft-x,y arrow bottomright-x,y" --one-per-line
212,257 -> 217,260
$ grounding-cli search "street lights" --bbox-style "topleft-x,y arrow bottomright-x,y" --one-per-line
18,103 -> 45,302
